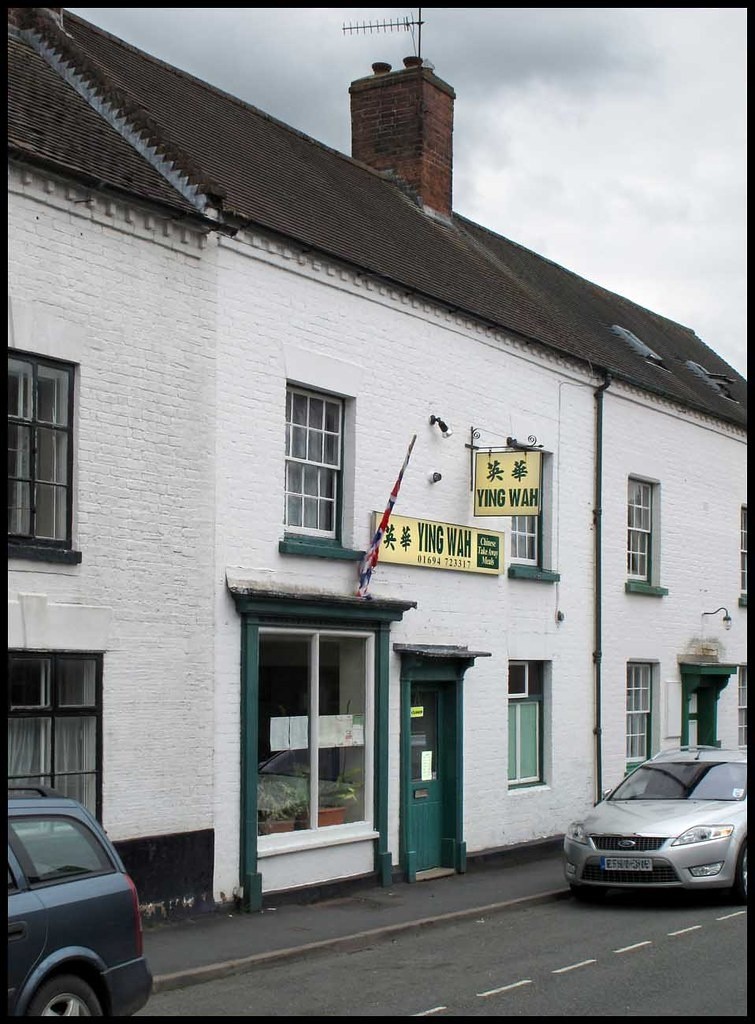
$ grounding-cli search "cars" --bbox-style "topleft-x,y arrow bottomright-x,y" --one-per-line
255,742 -> 340,817
564,744 -> 748,907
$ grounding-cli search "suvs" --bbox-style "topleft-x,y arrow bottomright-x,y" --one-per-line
7,783 -> 154,1017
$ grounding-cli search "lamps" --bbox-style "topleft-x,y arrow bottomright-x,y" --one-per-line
702,607 -> 731,630
430,414 -> 453,439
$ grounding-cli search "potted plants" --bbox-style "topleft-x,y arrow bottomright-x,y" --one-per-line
298,766 -> 364,829
257,768 -> 311,835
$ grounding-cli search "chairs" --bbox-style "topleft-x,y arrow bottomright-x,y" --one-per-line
644,765 -> 687,797
696,766 -> 743,798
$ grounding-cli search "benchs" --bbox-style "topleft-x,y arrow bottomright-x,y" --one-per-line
20,831 -> 104,870
